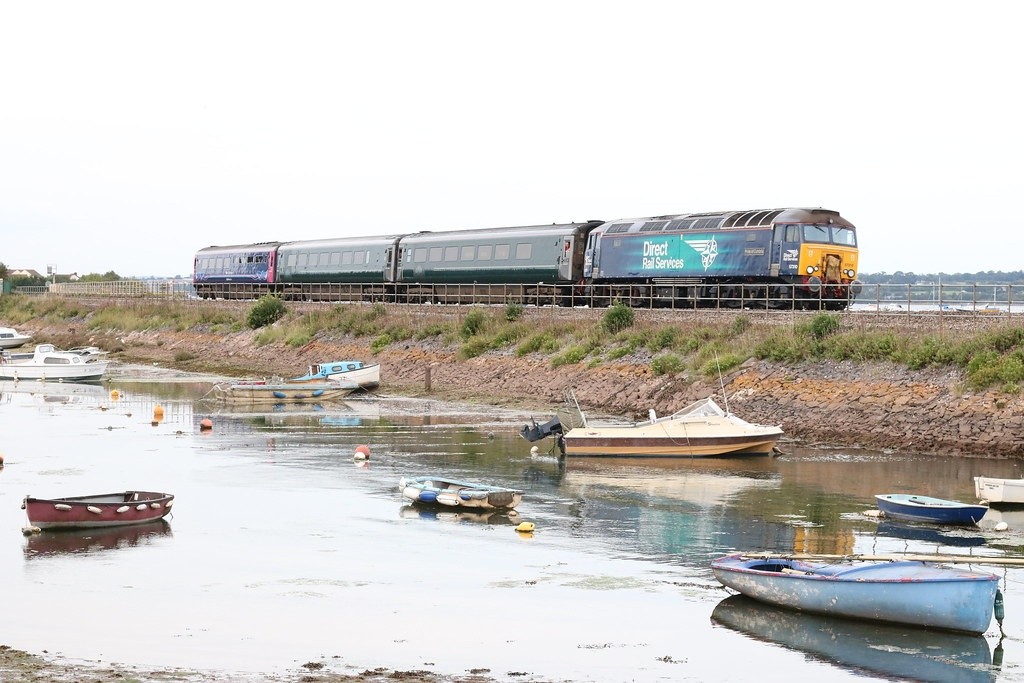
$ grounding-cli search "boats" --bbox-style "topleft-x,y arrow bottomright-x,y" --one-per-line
0,326 -> 34,349
712,552 -> 1003,638
705,593 -> 1003,683
288,361 -> 381,388
10,343 -> 108,362
24,489 -> 176,530
211,378 -> 361,404
973,476 -> 1024,503
874,493 -> 991,527
397,474 -> 526,513
0,341 -> 112,381
518,349 -> 785,459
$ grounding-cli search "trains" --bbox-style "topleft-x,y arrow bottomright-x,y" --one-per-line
192,205 -> 863,310
